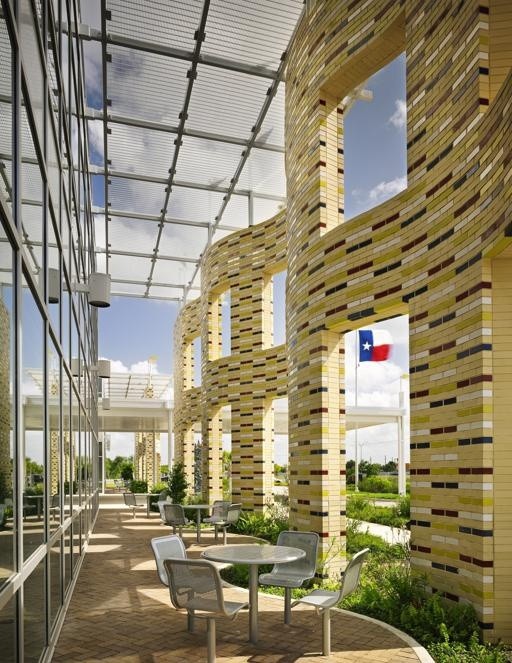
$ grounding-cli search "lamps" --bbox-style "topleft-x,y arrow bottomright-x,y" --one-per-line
39,268 -> 60,303
66,272 -> 111,311
97,396 -> 111,411
72,357 -> 84,376
87,358 -> 111,380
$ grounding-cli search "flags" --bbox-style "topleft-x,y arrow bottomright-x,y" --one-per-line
359,328 -> 394,361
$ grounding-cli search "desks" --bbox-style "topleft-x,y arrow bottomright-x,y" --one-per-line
136,491 -> 159,519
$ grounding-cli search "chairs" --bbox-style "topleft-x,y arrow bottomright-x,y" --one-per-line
122,491 -> 144,516
149,488 -> 371,662
